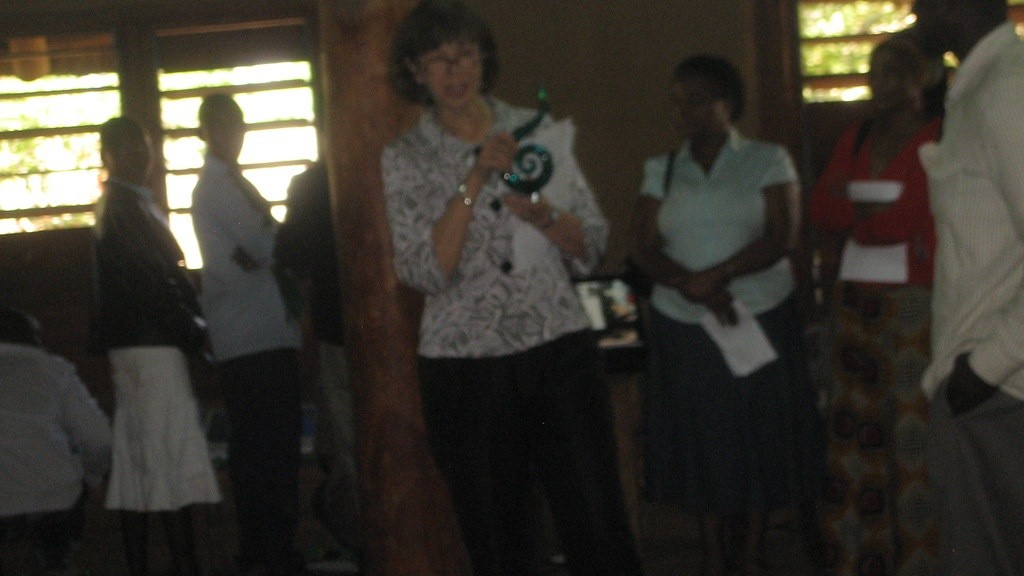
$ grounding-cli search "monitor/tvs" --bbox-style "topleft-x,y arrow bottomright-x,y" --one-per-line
572,271 -> 646,374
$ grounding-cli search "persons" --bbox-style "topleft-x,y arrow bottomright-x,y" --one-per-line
635,0 -> 1024,576
380,0 -> 641,576
0,94 -> 379,576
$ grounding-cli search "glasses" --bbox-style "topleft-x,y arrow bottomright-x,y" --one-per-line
417,50 -> 477,77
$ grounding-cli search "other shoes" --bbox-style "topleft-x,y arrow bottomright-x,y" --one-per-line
549,551 -> 567,565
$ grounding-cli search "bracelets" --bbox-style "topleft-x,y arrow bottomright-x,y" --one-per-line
541,209 -> 562,227
457,183 -> 473,206
